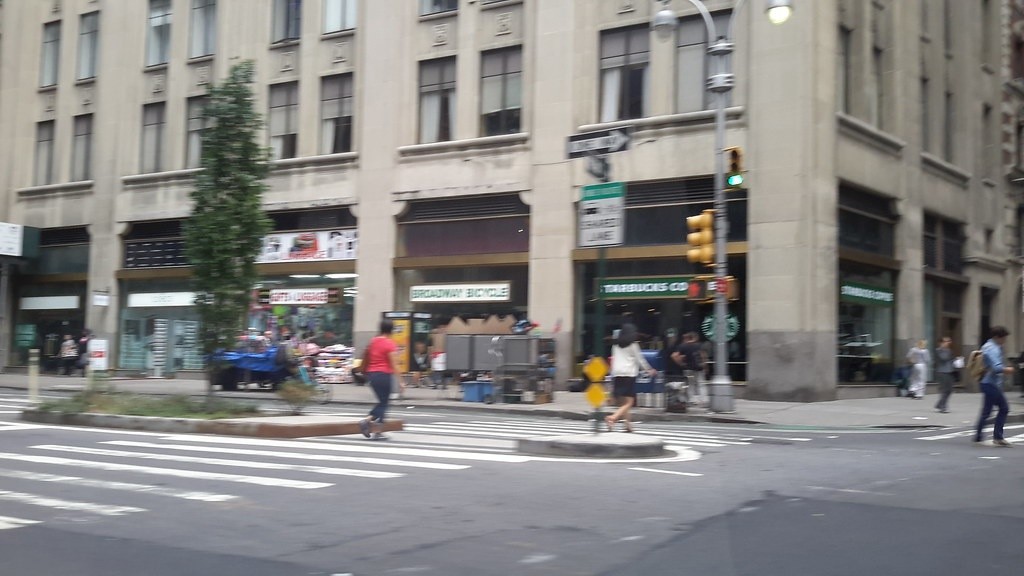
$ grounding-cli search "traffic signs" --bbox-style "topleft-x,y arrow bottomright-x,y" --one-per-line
565,124 -> 634,160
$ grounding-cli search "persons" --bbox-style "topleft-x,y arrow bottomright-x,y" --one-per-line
972,325 -> 1016,446
57,334 -> 80,375
358,318 -> 406,440
671,332 -> 710,405
934,335 -> 963,413
604,322 -> 657,434
906,339 -> 931,399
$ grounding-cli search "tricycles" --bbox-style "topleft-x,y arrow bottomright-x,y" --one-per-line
209,350 -> 333,407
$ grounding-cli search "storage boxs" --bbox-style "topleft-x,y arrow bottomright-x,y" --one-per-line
462,381 -> 494,402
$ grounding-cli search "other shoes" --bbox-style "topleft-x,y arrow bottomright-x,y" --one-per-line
625,424 -> 632,434
372,432 -> 388,440
934,406 -> 950,414
993,437 -> 1011,446
605,416 -> 615,431
359,419 -> 370,438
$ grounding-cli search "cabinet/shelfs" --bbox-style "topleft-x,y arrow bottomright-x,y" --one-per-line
314,348 -> 355,383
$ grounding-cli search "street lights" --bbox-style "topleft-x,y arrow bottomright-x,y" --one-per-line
649,0 -> 794,415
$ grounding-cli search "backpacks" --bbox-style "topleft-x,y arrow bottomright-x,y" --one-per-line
966,346 -> 996,381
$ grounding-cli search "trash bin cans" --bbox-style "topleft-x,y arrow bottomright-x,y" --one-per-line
666,382 -> 688,413
634,349 -> 666,408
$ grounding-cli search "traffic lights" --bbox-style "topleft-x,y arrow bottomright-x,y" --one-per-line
729,143 -> 753,188
685,209 -> 715,267
